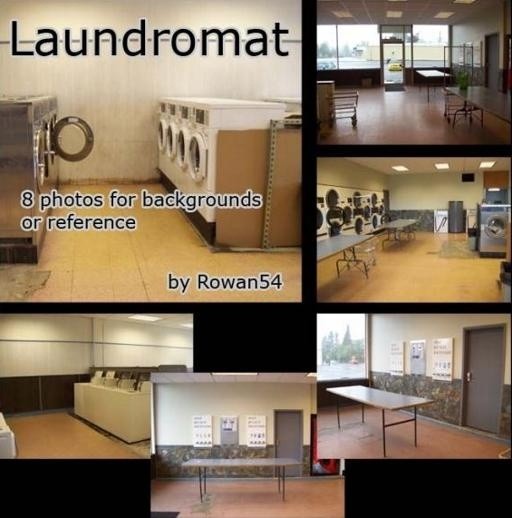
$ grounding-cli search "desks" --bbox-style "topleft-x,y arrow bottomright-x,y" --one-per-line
317,218 -> 418,280
180,457 -> 302,501
325,385 -> 434,457
416,70 -> 511,129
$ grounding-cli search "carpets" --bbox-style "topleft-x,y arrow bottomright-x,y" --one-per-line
385,83 -> 406,92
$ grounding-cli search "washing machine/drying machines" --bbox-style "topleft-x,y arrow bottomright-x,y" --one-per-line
316,181 -> 387,243
476,203 -> 511,254
0,93 -> 95,264
154,96 -> 289,253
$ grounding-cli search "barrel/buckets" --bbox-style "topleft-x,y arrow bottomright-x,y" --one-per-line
448,200 -> 464,233
